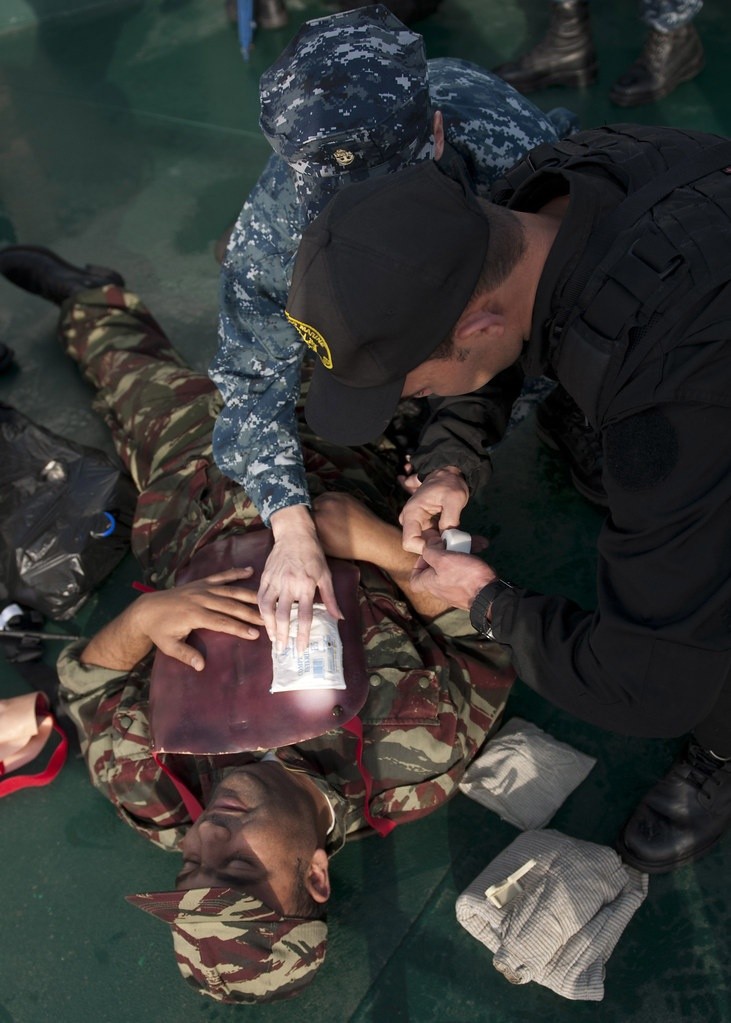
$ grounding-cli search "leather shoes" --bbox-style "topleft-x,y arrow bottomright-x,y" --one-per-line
535,389 -> 612,507
617,735 -> 731,874
0,244 -> 124,307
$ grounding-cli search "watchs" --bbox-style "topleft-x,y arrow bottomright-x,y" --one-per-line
470,577 -> 514,641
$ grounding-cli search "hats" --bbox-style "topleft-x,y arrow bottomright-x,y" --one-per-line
284,159 -> 488,447
258,2 -> 432,237
125,886 -> 330,1006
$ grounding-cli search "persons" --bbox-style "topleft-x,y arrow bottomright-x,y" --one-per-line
211,5 -> 574,653
0,240 -> 514,1006
287,122 -> 731,877
493,0 -> 706,107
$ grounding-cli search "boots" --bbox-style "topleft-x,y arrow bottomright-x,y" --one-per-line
610,19 -> 705,107
494,0 -> 599,94
